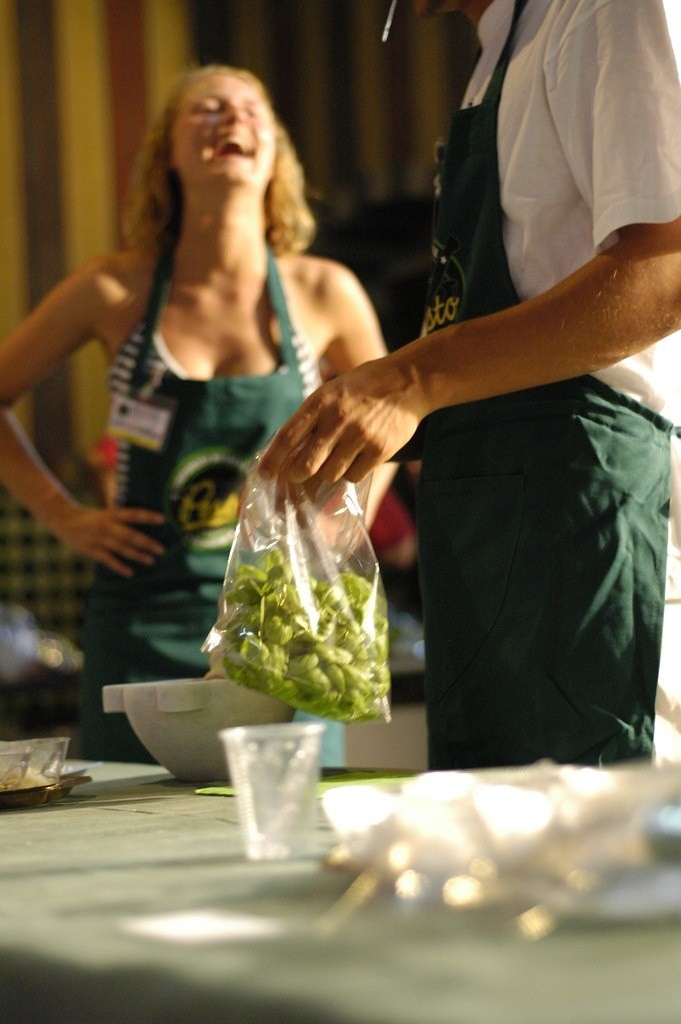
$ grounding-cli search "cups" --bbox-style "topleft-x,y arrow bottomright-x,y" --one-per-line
217,720 -> 326,863
0,736 -> 72,791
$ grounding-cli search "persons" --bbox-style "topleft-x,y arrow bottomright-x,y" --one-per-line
256,0 -> 679,772
0,62 -> 392,764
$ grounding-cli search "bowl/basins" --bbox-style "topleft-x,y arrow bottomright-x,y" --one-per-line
101,679 -> 294,783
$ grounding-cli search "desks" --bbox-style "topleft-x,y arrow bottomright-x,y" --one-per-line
1,760 -> 681,1024
